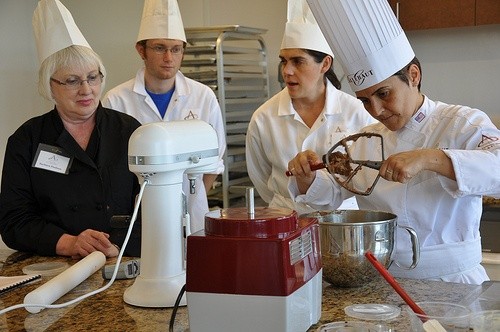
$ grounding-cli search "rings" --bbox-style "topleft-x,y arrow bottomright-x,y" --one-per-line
290,167 -> 295,172
386,169 -> 393,174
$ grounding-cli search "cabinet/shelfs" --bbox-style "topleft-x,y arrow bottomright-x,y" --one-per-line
388,0 -> 500,31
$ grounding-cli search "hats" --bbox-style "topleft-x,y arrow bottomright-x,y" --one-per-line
135,0 -> 187,44
31,0 -> 92,64
279,0 -> 334,58
306,0 -> 414,92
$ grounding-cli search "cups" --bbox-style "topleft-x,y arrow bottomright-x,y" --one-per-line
317,321 -> 389,332
405,301 -> 471,332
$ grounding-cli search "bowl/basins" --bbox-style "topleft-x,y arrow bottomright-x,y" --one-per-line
300,209 -> 419,288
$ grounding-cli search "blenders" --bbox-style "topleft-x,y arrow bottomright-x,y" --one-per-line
122,121 -> 225,306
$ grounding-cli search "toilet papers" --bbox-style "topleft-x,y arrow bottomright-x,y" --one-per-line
23,251 -> 106,314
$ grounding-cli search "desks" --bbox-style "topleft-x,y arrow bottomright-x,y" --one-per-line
0,255 -> 500,332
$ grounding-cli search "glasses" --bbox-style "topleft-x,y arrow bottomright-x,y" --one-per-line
144,45 -> 184,54
50,71 -> 104,90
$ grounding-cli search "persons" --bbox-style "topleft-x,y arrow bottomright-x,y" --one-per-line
287,0 -> 500,286
102,0 -> 226,196
0,0 -> 142,259
246,0 -> 380,209
